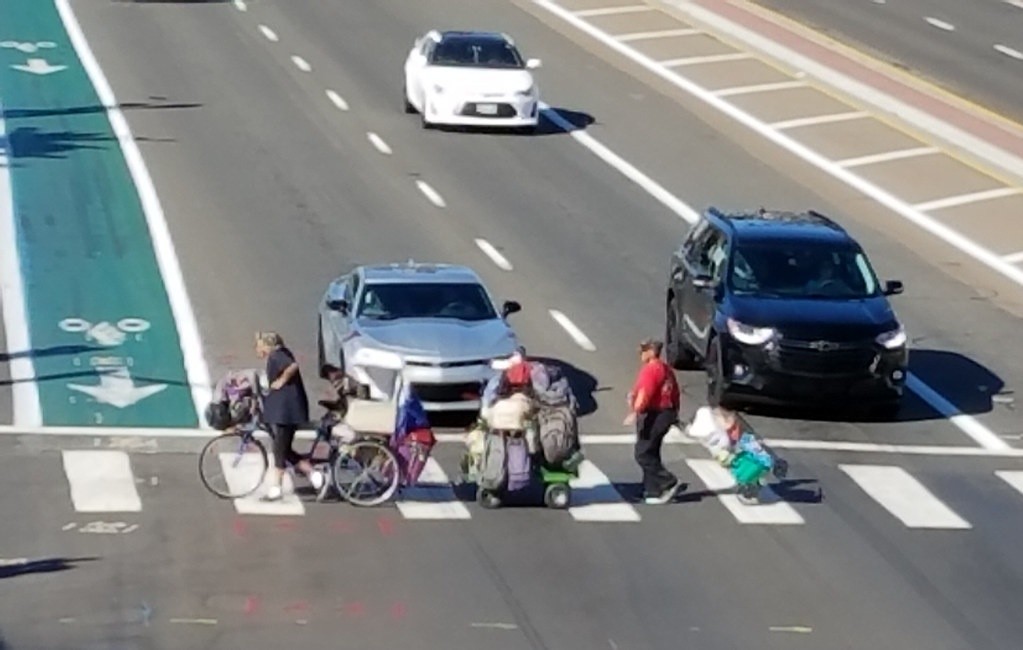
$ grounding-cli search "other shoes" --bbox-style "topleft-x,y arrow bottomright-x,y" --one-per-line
259,494 -> 282,502
314,472 -> 330,501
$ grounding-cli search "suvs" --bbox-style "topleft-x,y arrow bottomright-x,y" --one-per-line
664,206 -> 908,419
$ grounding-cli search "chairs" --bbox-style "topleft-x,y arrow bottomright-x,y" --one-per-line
734,248 -> 785,291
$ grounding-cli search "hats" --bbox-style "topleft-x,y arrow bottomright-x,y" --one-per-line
640,339 -> 664,349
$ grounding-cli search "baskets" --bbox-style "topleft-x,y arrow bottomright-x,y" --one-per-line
220,399 -> 252,427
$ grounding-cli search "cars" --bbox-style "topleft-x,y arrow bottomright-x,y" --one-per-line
316,264 -> 523,413
403,30 -> 541,134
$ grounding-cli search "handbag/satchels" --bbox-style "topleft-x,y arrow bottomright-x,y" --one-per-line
205,402 -> 232,431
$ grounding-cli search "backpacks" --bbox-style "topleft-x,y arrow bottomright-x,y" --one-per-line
476,433 -> 507,490
507,434 -> 532,492
539,405 -> 579,465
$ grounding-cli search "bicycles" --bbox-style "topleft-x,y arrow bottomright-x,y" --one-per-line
198,388 -> 402,507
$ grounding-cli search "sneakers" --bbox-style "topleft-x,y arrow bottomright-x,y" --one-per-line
636,493 -> 660,504
659,479 -> 688,503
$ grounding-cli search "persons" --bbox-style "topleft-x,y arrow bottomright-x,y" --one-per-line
622,338 -> 685,505
253,331 -> 333,505
